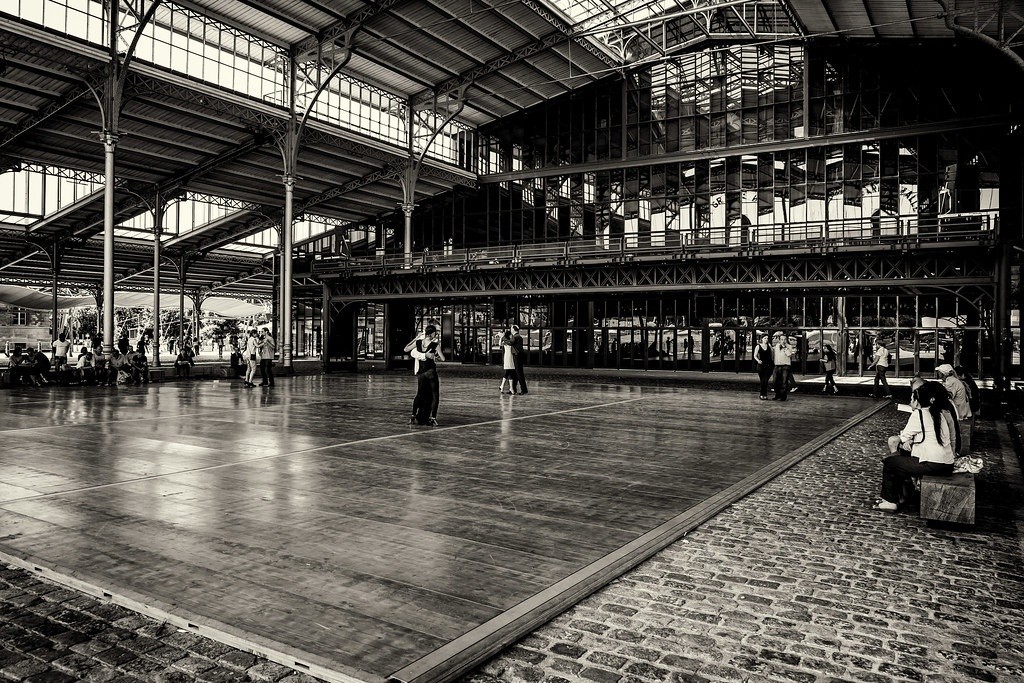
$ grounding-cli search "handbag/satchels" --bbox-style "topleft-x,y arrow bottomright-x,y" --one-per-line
887,354 -> 892,364
750,360 -> 762,372
898,439 -> 913,456
251,354 -> 257,360
824,361 -> 835,371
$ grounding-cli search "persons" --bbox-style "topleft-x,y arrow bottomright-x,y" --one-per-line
3,325 -> 280,388
497,323 -> 528,396
404,319 -> 446,426
658,320 -> 980,511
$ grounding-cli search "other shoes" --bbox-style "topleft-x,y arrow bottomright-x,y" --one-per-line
771,389 -> 774,392
883,395 -> 893,399
871,499 -> 898,513
790,387 -> 798,393
772,396 -> 787,401
819,391 -> 827,395
833,390 -> 839,395
268,382 -> 274,387
869,394 -> 877,398
259,381 -> 268,386
518,390 -> 528,395
409,416 -> 438,427
760,396 -> 768,400
506,391 -> 512,394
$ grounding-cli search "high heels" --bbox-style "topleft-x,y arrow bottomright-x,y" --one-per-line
249,382 -> 256,387
509,389 -> 517,395
497,386 -> 505,394
244,381 -> 250,386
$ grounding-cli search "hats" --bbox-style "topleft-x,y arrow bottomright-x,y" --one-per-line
935,364 -> 955,376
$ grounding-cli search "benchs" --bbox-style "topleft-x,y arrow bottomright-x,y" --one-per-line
921,413 -> 977,524
